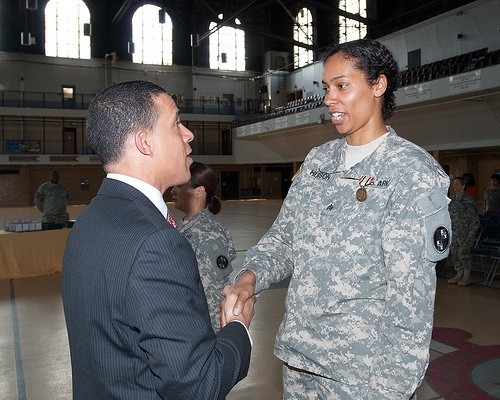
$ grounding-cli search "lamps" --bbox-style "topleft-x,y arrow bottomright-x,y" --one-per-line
128,41 -> 134,54
191,33 -> 199,47
21,32 -> 30,45
159,10 -> 166,23
26,0 -> 38,11
84,23 -> 91,36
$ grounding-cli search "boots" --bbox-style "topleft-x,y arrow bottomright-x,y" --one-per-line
448,268 -> 464,284
458,268 -> 471,286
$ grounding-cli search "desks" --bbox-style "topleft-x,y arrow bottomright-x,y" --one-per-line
0,227 -> 72,281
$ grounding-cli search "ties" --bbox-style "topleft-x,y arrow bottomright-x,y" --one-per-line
165,209 -> 177,230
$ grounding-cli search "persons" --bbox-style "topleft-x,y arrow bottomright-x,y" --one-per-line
220,39 -> 453,400
34,169 -> 72,220
60,80 -> 257,400
447,172 -> 500,286
171,161 -> 236,334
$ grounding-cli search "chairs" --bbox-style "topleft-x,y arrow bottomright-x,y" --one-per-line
436,210 -> 500,286
230,47 -> 500,127
